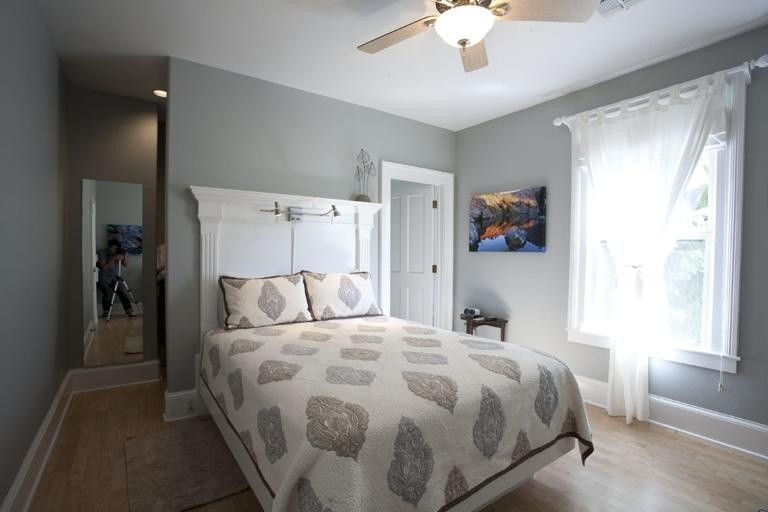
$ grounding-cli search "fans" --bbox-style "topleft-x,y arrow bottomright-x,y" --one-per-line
353,0 -> 604,74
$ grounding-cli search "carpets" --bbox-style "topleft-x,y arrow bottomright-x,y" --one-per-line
122,415 -> 252,511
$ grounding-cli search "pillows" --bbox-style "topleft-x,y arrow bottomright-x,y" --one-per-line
217,268 -> 381,330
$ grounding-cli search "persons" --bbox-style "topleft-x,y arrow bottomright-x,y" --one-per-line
96,238 -> 137,318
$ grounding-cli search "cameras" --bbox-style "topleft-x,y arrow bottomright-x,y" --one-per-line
114,247 -> 127,255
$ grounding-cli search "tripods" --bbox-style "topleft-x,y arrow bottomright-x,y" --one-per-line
107,256 -> 144,323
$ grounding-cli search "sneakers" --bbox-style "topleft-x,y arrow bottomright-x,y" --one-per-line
128,310 -> 137,318
103,311 -> 110,319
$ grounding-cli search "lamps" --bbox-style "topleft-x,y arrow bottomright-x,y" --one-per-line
263,201 -> 342,219
437,4 -> 494,50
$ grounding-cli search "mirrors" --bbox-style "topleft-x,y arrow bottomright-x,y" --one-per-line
80,174 -> 148,372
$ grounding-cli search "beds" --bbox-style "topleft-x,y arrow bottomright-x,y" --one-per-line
185,184 -> 595,511
